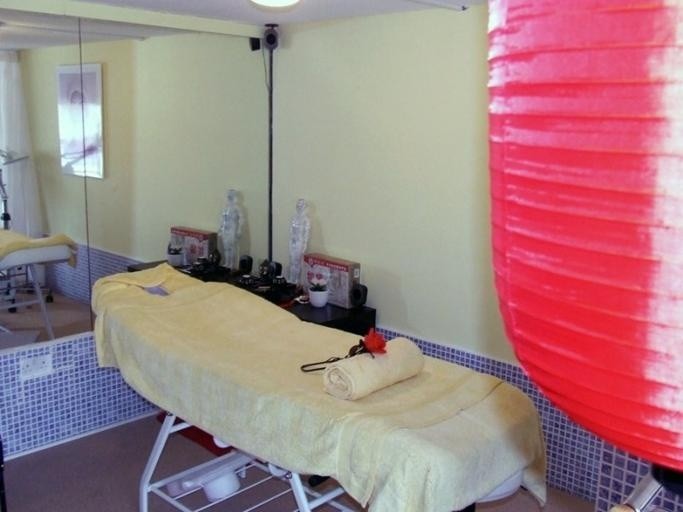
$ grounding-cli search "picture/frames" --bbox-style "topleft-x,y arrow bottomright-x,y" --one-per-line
54,63 -> 105,179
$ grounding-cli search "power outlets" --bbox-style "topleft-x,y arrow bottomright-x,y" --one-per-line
19,353 -> 54,380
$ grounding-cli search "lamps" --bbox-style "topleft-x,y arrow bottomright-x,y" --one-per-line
0,150 -> 30,230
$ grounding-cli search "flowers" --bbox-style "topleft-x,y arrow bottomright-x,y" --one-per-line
300,328 -> 387,373
307,271 -> 326,290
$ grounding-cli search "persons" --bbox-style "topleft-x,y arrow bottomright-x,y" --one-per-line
287,199 -> 310,283
218,188 -> 244,268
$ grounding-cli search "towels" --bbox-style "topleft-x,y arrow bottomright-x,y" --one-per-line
322,337 -> 424,401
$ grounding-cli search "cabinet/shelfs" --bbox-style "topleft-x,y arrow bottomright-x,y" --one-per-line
124,259 -> 260,284
222,274 -> 376,336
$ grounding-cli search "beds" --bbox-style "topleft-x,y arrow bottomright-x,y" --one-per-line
88,261 -> 547,512
0,229 -> 79,339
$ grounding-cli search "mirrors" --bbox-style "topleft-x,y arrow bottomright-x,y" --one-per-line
0,8 -> 274,352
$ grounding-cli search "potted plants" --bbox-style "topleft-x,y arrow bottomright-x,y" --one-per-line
307,288 -> 329,308
